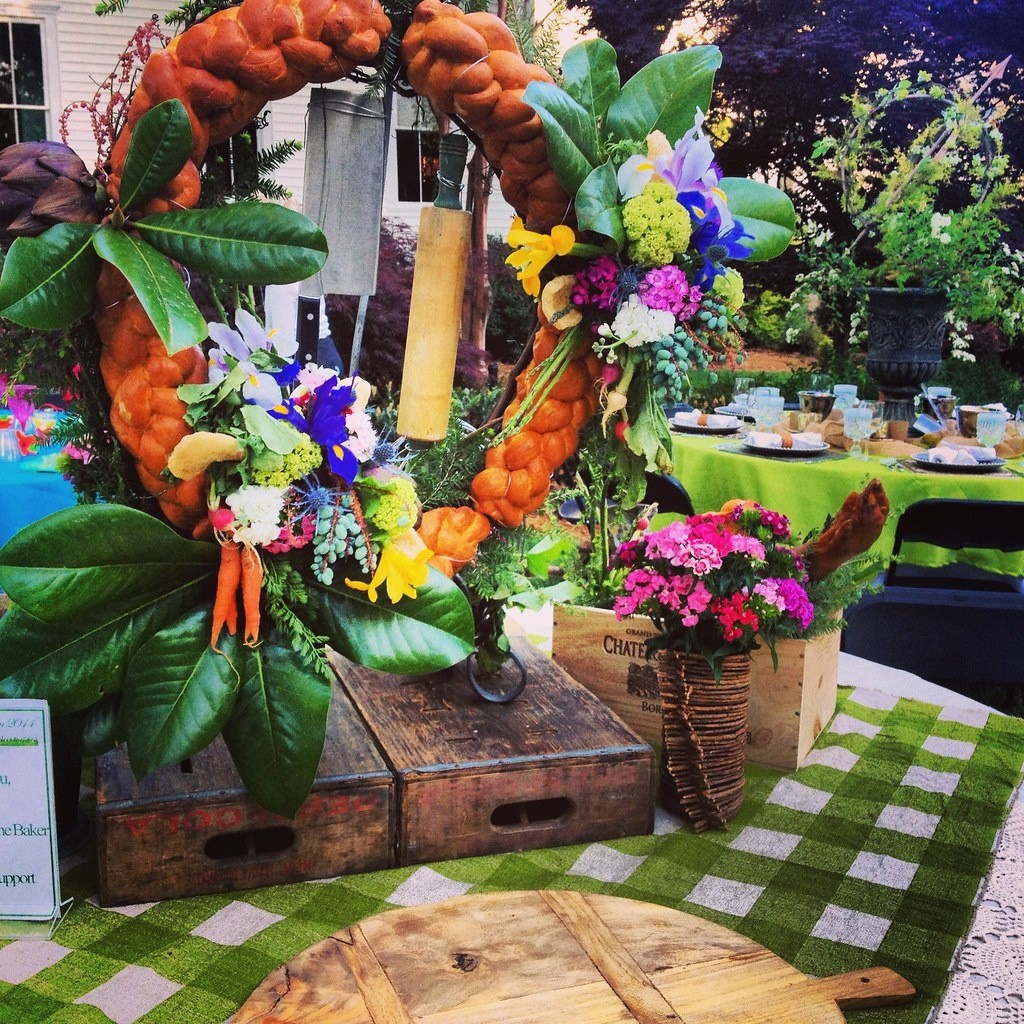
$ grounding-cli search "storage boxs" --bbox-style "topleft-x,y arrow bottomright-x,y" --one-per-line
553,596 -> 844,774
322,642 -> 657,868
95,663 -> 397,910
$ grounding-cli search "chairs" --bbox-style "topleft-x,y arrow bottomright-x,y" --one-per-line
839,586 -> 1024,718
607,471 -> 696,517
885,498 -> 1024,593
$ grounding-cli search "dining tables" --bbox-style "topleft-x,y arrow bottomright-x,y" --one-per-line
671,410 -> 1024,576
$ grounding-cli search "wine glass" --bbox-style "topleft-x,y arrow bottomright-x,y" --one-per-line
859,400 -> 886,462
977,412 -> 1006,449
844,408 -> 873,456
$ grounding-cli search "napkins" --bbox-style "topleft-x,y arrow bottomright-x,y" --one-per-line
928,441 -> 996,465
745,431 -> 823,449
674,411 -> 738,428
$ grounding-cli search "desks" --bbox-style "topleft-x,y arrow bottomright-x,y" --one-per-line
0,647 -> 1024,1024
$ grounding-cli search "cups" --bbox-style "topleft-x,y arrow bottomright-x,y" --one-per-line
834,384 -> 857,408
928,387 -> 951,396
751,397 -> 785,430
889,421 -> 909,439
747,386 -> 780,409
875,421 -> 889,438
731,378 -> 755,407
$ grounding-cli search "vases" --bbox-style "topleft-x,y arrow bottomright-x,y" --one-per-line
857,287 -> 951,439
648,648 -> 749,834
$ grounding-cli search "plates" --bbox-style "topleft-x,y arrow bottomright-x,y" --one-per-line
911,451 -> 1007,471
714,406 -> 751,419
743,437 -> 830,452
668,417 -> 744,431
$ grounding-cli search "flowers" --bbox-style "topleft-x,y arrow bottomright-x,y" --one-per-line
0,307 -> 477,821
503,38 -> 1024,471
610,498 -> 815,685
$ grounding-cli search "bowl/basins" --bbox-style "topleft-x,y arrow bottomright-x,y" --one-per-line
955,406 -> 1014,438
919,395 -> 956,424
798,391 -> 837,422
